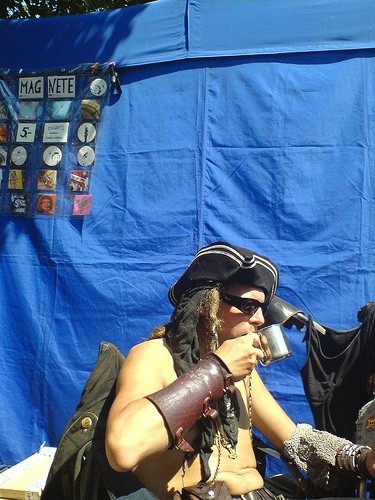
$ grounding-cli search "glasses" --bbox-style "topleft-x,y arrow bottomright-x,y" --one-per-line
219,293 -> 271,316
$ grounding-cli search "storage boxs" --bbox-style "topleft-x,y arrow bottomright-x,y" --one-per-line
1,448 -> 58,499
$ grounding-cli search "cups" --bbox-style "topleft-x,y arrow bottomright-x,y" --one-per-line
257,323 -> 292,366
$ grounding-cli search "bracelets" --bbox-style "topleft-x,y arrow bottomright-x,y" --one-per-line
337,444 -> 372,471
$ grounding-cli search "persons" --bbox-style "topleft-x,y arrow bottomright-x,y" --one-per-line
105,241 -> 375,500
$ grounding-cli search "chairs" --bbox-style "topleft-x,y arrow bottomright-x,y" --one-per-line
68,341 -> 367,500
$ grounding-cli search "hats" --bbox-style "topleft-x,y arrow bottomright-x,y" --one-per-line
168,241 -> 280,308
